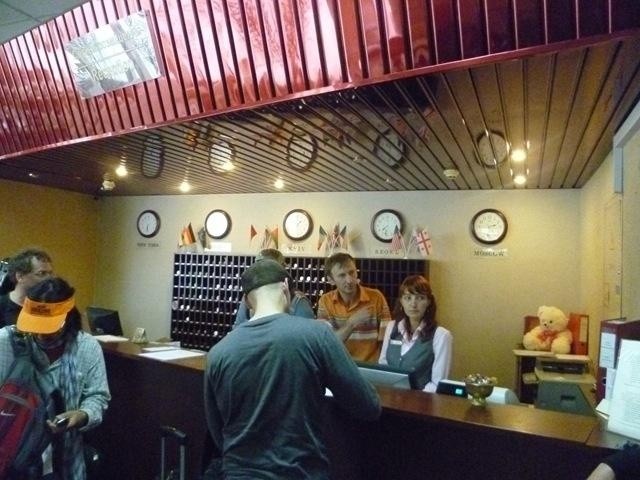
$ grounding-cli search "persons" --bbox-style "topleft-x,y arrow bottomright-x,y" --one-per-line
0,248 -> 82,332
376,275 -> 454,393
585,447 -> 640,480
316,252 -> 393,364
203,259 -> 382,479
0,257 -> 12,289
0,275 -> 112,480
226,248 -> 316,330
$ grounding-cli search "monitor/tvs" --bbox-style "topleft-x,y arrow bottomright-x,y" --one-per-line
356,360 -> 418,390
85,307 -> 124,336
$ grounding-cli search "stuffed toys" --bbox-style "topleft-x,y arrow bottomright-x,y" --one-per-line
523,305 -> 574,354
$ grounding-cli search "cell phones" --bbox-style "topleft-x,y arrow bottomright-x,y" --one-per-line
57,416 -> 69,427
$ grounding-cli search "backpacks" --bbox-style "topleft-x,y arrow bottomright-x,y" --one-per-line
0,324 -> 66,479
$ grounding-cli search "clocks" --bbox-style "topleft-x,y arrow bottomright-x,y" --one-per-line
474,131 -> 513,170
141,135 -> 166,178
373,129 -> 406,168
137,209 -> 162,238
205,141 -> 235,176
471,207 -> 508,245
282,208 -> 313,240
286,132 -> 319,173
204,209 -> 232,239
369,209 -> 404,243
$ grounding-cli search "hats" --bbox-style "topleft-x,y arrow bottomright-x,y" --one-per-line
16,291 -> 77,334
241,260 -> 293,294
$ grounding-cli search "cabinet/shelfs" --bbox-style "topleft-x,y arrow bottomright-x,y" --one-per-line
168,251 -> 430,353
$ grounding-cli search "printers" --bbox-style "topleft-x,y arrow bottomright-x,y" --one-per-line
521,357 -> 598,418
